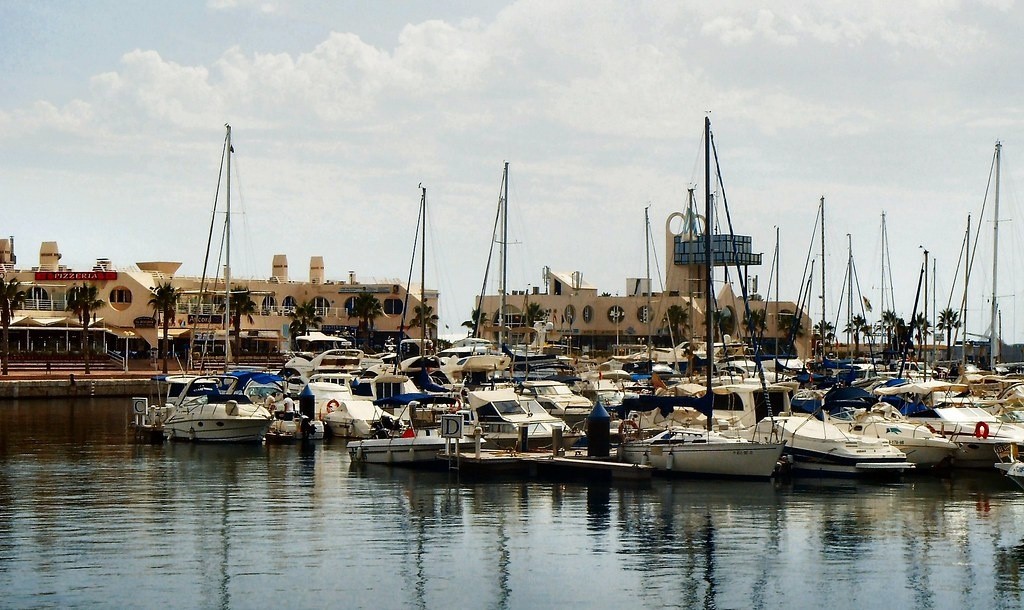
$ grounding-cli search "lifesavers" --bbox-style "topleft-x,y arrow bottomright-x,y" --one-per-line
975,421 -> 990,439
618,420 -> 640,441
326,400 -> 339,413
450,397 -> 462,412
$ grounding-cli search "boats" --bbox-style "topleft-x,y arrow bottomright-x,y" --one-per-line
894,406 -> 1024,463
232,348 -> 403,440
810,402 -> 958,473
745,410 -> 917,486
346,400 -> 487,467
442,392 -> 586,451
130,376 -> 275,444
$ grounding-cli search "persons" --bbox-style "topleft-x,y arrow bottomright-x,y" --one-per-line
266,392 -> 277,410
282,394 -> 295,421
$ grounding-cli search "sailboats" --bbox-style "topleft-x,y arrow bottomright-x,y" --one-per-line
153,124 -> 266,410
378,183 -> 472,406
449,159 -> 535,394
599,110 -> 1024,481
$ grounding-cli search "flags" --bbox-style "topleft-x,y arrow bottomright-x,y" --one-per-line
862,295 -> 873,312
